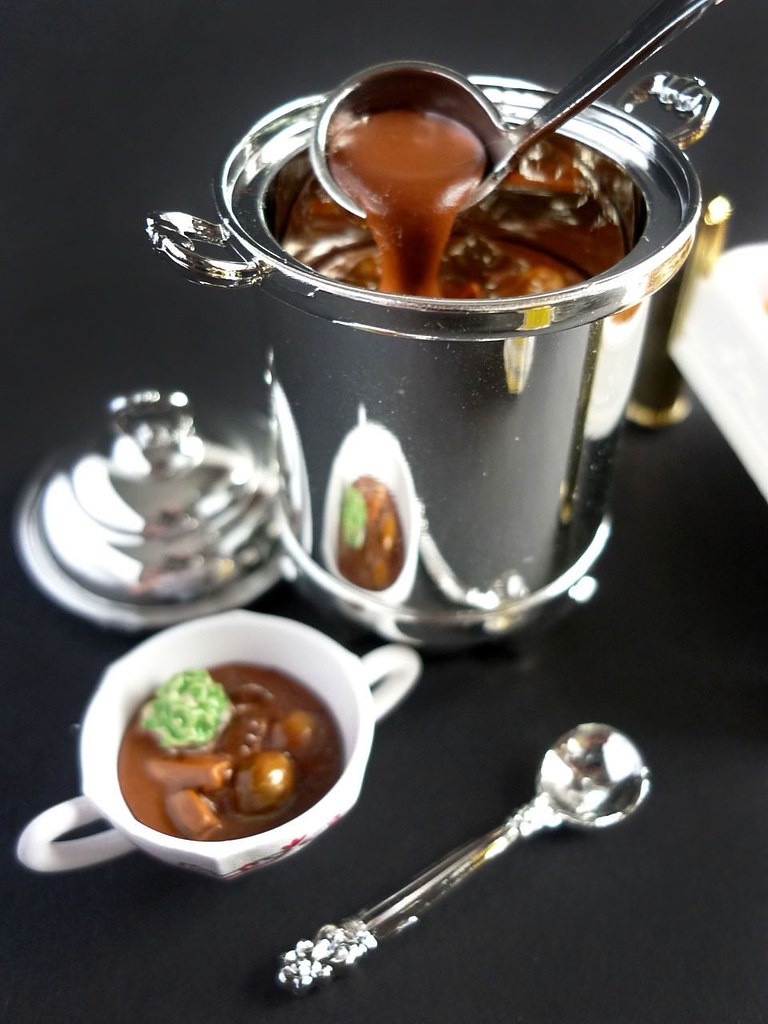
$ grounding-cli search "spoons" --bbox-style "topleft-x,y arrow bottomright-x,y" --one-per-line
276,723 -> 652,994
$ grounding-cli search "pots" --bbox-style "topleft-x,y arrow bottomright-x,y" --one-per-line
142,71 -> 720,649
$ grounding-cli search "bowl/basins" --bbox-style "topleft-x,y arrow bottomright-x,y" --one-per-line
319,401 -> 427,644
14,609 -> 421,877
